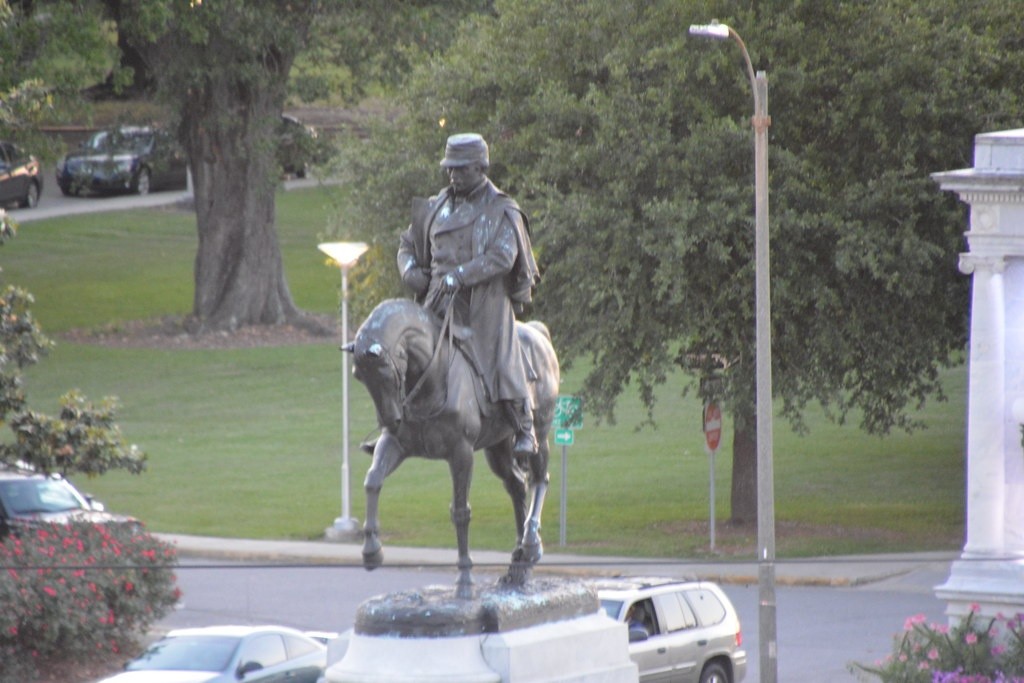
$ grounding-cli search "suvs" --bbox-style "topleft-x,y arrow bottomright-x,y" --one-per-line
584,570 -> 748,683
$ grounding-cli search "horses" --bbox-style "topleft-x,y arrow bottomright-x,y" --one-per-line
339,297 -> 559,599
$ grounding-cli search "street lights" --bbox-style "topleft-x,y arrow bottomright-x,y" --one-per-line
311,240 -> 373,544
690,19 -> 779,683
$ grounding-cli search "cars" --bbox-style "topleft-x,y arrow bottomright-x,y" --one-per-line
0,139 -> 45,208
98,624 -> 329,683
0,459 -> 139,537
274,114 -> 318,178
54,124 -> 188,198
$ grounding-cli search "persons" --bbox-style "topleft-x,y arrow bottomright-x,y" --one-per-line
624,608 -> 641,632
359,134 -> 542,452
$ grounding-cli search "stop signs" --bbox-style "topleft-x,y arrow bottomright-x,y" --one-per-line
703,401 -> 722,451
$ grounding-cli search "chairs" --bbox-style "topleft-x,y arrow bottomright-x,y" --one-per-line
632,607 -> 645,623
